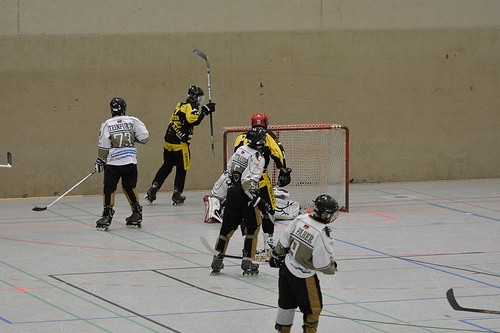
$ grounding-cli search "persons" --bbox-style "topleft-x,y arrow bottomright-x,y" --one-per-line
95,97 -> 149,231
269,194 -> 340,333
204,187 -> 302,223
234,112 -> 292,261
210,127 -> 276,276
145,85 -> 216,206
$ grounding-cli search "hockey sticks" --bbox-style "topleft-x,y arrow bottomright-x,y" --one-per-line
32,170 -> 97,211
200,236 -> 270,264
0,152 -> 13,168
446,288 -> 500,314
192,48 -> 215,157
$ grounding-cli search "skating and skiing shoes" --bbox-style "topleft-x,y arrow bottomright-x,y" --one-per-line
241,260 -> 260,275
210,256 -> 225,275
95,208 -> 115,230
125,206 -> 142,228
263,233 -> 275,261
172,192 -> 186,206
255,249 -> 266,261
145,186 -> 157,204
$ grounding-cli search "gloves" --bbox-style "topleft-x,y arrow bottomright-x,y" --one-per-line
201,103 -> 216,115
252,195 -> 275,215
276,168 -> 292,187
94,158 -> 106,173
269,251 -> 285,268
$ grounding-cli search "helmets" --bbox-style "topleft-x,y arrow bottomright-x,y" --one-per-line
110,97 -> 126,117
246,127 -> 266,148
251,113 -> 268,129
187,86 -> 204,102
313,194 -> 338,224
